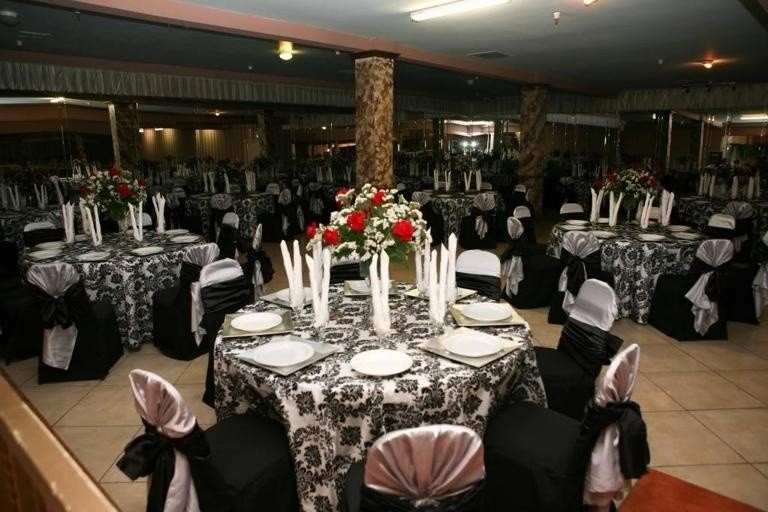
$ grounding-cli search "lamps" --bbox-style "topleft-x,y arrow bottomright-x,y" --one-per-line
701,59 -> 714,69
409,0 -> 510,22
277,41 -> 293,61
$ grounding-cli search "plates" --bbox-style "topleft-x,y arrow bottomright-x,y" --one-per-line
27,228 -> 198,263
681,195 -> 768,205
562,218 -> 699,243
444,332 -> 504,359
349,282 -> 393,293
278,286 -> 317,302
422,189 -> 478,198
252,340 -> 315,368
461,302 -> 511,322
188,190 -> 273,199
231,311 -> 283,332
350,350 -> 414,376
